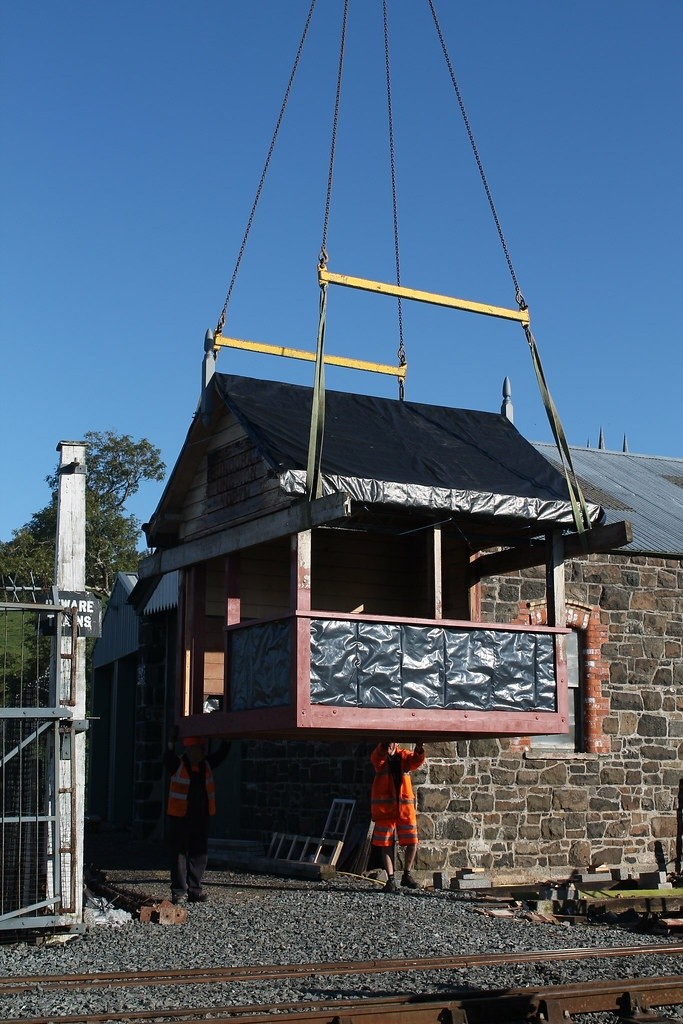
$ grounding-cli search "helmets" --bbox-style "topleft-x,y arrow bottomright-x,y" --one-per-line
183,736 -> 204,747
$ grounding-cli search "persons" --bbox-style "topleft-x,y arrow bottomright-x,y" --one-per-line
371,740 -> 426,890
167,729 -> 232,903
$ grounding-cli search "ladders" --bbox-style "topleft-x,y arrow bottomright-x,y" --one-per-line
267,832 -> 345,867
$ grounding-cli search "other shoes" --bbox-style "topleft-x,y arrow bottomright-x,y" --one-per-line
187,893 -> 211,902
400,875 -> 421,889
385,880 -> 396,893
172,893 -> 186,904
